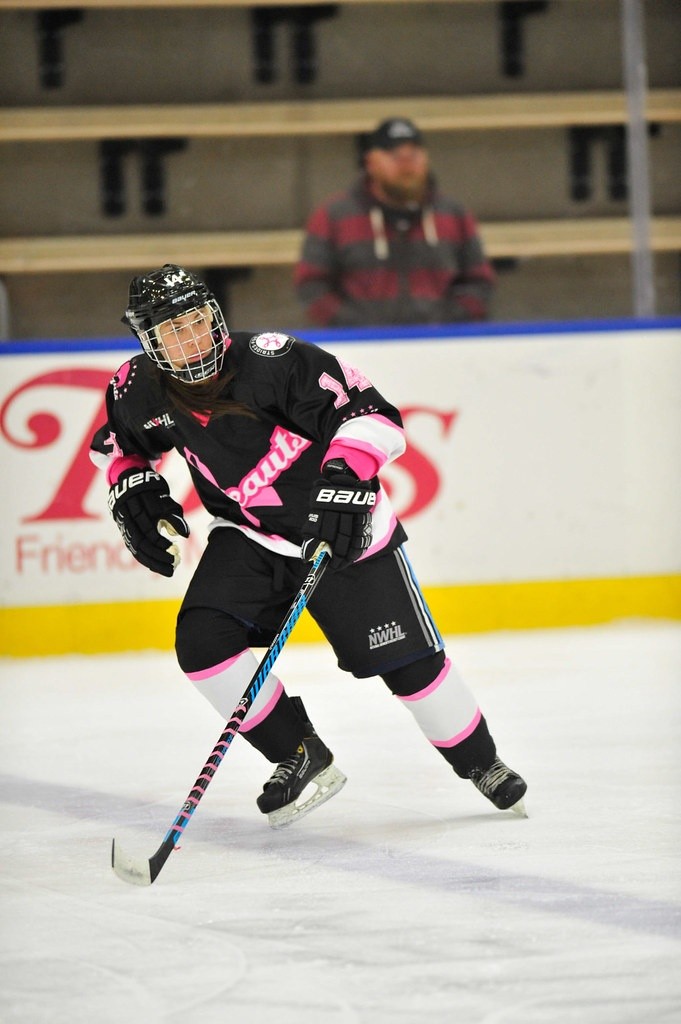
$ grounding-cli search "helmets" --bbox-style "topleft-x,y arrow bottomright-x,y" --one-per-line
120,263 -> 229,384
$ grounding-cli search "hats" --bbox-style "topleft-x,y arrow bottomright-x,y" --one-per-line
361,116 -> 423,147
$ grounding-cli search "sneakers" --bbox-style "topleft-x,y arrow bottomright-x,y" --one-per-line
256,722 -> 347,830
470,753 -> 529,818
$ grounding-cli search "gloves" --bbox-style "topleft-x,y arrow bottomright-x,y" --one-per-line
302,458 -> 380,573
106,467 -> 190,577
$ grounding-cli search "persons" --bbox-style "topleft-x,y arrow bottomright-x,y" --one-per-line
295,120 -> 494,327
90,264 -> 527,827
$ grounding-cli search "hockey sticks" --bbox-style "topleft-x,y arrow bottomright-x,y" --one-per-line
106,542 -> 332,888
